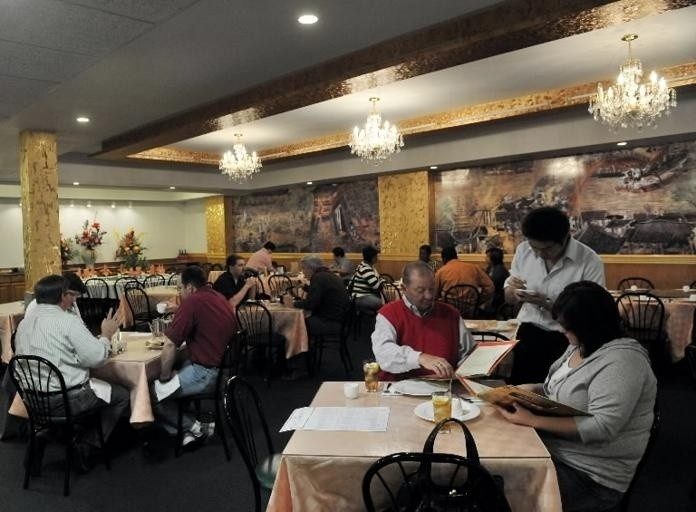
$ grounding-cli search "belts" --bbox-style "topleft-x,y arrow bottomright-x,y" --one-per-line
24,383 -> 82,397
194,362 -> 219,369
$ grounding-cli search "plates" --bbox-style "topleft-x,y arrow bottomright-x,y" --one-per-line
631,297 -> 656,302
626,289 -> 648,293
392,378 -> 448,396
146,345 -> 164,349
675,289 -> 696,302
414,398 -> 481,423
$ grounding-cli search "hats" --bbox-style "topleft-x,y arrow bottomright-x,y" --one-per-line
362,246 -> 378,256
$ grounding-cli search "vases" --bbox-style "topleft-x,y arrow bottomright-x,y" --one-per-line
127,255 -> 138,266
64,260 -> 69,270
81,249 -> 98,268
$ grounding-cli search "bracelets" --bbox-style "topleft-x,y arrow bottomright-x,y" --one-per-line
538,298 -> 550,313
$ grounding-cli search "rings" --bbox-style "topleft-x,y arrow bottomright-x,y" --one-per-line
437,363 -> 442,369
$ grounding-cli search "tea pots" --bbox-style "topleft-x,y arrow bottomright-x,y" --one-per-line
147,318 -> 172,337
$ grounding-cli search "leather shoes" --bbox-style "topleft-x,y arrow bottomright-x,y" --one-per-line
70,440 -> 92,473
281,370 -> 308,381
92,440 -> 108,465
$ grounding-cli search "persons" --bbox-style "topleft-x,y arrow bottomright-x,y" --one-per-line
246,242 -> 277,275
370,261 -> 477,380
502,206 -> 606,385
282,255 -> 352,381
432,246 -> 495,320
212,254 -> 257,306
419,245 -> 443,273
148,266 -> 241,457
455,280 -> 657,511
485,248 -> 510,320
25,271 -> 86,319
328,247 -> 352,287
346,246 -> 383,309
15,274 -> 131,473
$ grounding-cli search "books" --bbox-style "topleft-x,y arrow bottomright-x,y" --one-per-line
456,375 -> 594,416
416,340 -> 521,381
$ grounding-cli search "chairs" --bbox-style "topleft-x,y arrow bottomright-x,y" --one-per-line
8,355 -> 108,495
624,342 -> 674,512
361,452 -> 496,512
223,374 -> 282,511
175,327 -> 249,457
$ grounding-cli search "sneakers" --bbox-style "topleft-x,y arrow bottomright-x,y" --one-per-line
182,432 -> 196,447
195,421 -> 215,445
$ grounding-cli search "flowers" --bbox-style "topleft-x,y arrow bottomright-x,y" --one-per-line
61,239 -> 80,260
116,230 -> 148,257
75,222 -> 107,250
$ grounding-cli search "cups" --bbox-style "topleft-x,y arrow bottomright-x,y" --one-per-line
343,382 -> 359,399
270,289 -> 277,303
431,391 -> 453,434
683,285 -> 696,300
362,359 -> 379,392
276,267 -> 283,275
630,285 -> 637,289
156,304 -> 167,313
640,295 -> 646,300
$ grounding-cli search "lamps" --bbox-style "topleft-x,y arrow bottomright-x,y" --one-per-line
348,97 -> 405,166
219,133 -> 263,184
588,34 -> 678,134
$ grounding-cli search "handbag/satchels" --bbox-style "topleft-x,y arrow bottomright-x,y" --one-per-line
392,416 -> 513,511
